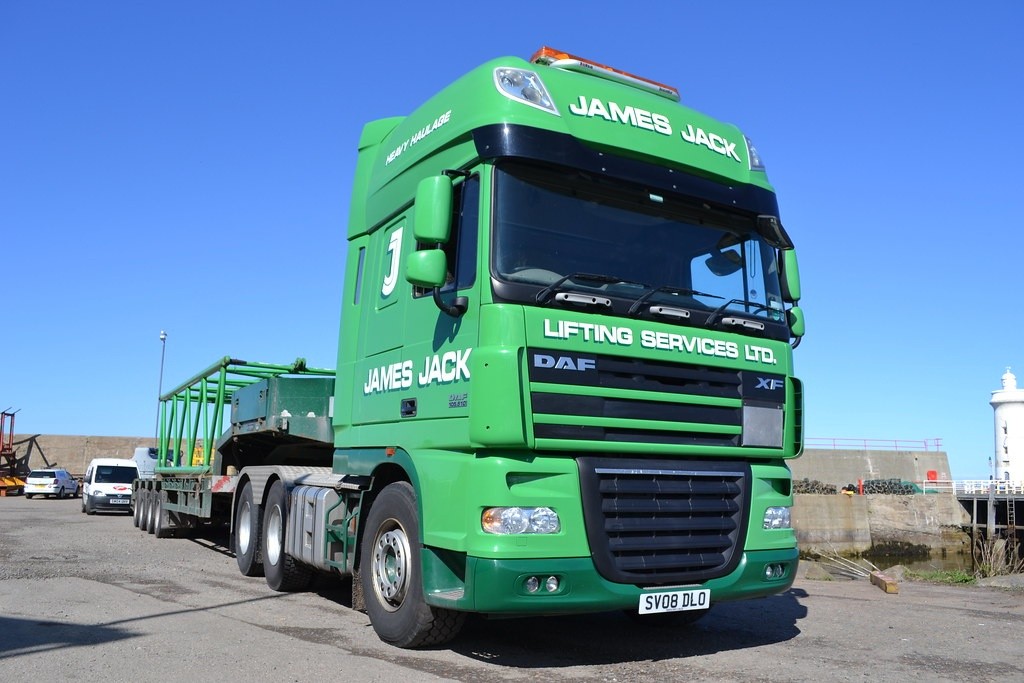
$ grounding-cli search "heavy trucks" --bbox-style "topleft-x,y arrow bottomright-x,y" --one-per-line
131,45 -> 808,647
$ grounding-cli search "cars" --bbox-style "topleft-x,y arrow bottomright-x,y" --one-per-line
23,467 -> 81,500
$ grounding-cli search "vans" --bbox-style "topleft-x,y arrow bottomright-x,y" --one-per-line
80,458 -> 140,514
